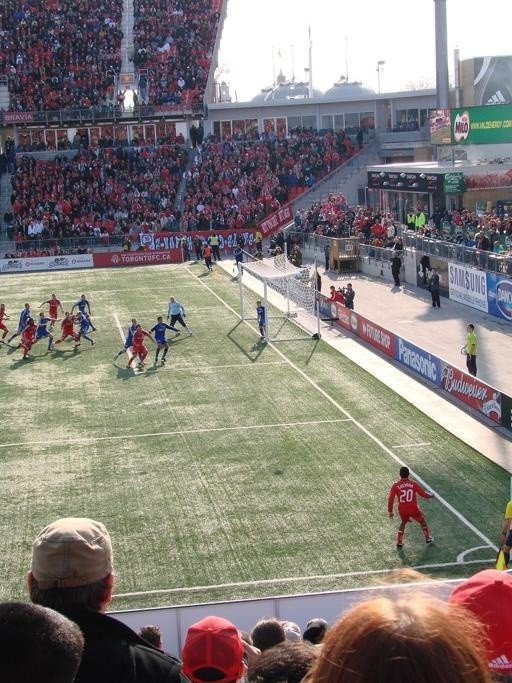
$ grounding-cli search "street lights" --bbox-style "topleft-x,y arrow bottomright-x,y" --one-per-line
377,61 -> 386,96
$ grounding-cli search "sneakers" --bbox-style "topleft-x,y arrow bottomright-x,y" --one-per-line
426,537 -> 434,544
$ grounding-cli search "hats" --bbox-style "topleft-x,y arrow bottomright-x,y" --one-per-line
182,615 -> 244,683
450,568 -> 512,672
32,517 -> 113,590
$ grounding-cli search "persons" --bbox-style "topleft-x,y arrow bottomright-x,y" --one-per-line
461,323 -> 478,377
148,317 -> 181,362
113,318 -> 147,365
315,271 -> 321,293
390,252 -> 401,286
2,504 -> 512,683
167,297 -> 193,337
1,1 -> 511,279
125,324 -> 156,368
0,294 -> 96,360
387,467 -> 435,546
426,268 -> 440,308
324,285 -> 345,305
256,300 -> 266,337
342,283 -> 355,310
